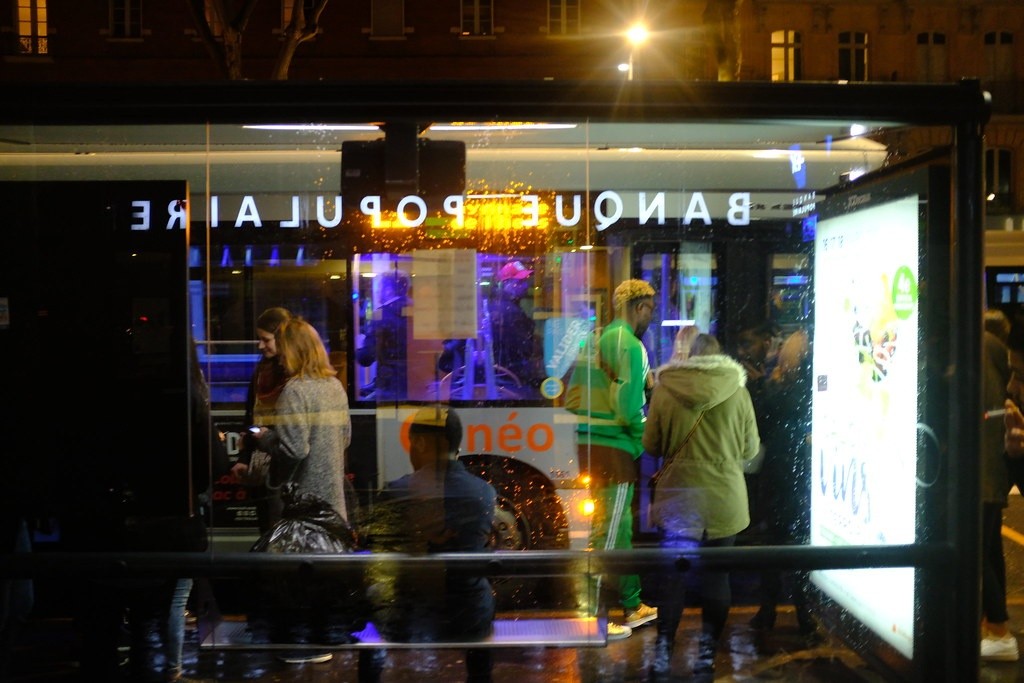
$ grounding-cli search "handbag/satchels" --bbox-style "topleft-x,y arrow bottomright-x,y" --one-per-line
241,490 -> 373,641
647,467 -> 662,505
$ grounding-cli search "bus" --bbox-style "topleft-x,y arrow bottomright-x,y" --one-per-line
185,184 -> 713,614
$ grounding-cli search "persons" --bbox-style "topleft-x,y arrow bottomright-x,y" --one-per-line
354,404 -> 497,683
1000,318 -> 1024,496
229,305 -> 292,633
69,329 -> 226,677
359,260 -> 536,402
981,330 -> 1019,662
563,277 -> 658,643
250,316 -> 352,664
732,313 -> 821,640
640,332 -> 762,682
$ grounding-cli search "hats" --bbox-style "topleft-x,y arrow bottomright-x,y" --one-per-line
410,405 -> 463,437
497,261 -> 534,280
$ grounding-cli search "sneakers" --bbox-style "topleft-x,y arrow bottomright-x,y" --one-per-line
625,602 -> 657,629
582,616 -> 632,640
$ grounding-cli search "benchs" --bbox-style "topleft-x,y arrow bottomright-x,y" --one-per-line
199,611 -> 609,653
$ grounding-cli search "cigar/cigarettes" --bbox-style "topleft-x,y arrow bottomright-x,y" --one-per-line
983,407 -> 1013,421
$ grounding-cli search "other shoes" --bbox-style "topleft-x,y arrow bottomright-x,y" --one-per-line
693,633 -> 715,683
799,619 -> 818,638
650,634 -> 673,683
276,641 -> 333,663
750,610 -> 776,632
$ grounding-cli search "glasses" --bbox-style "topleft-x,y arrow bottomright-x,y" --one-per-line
635,302 -> 654,314
